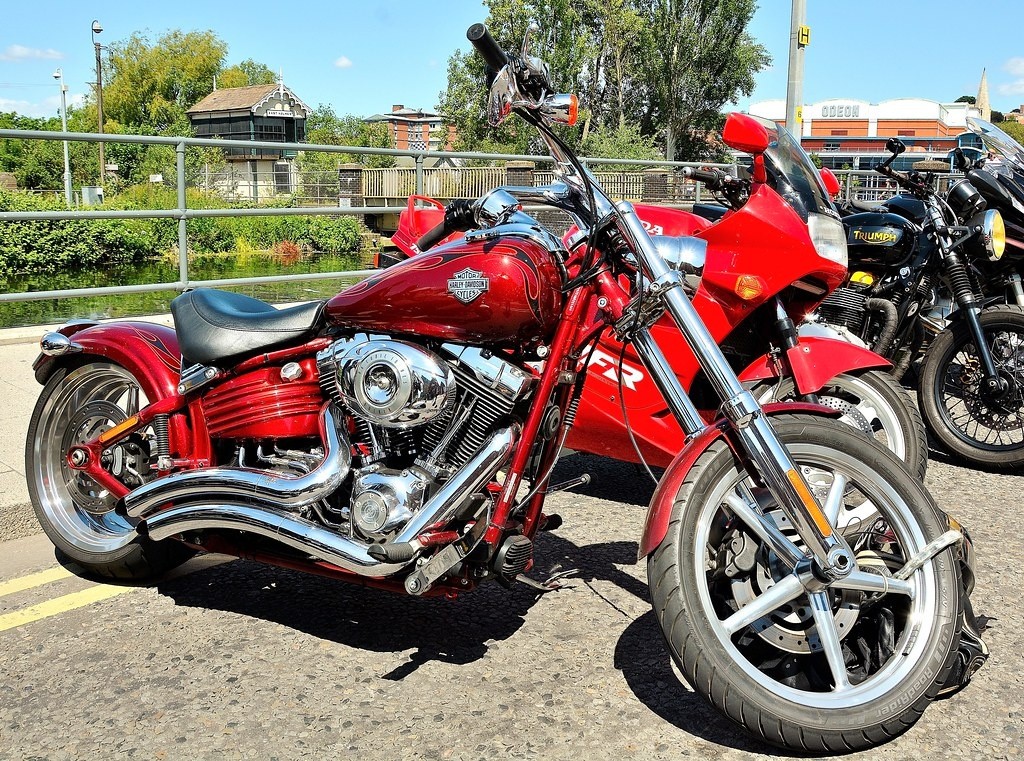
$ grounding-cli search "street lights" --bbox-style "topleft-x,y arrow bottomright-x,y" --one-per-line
92,18 -> 107,187
54,67 -> 72,203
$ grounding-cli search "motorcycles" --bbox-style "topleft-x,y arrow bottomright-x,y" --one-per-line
24,12 -> 991,756
367,112 -> 929,545
687,109 -> 1024,477
